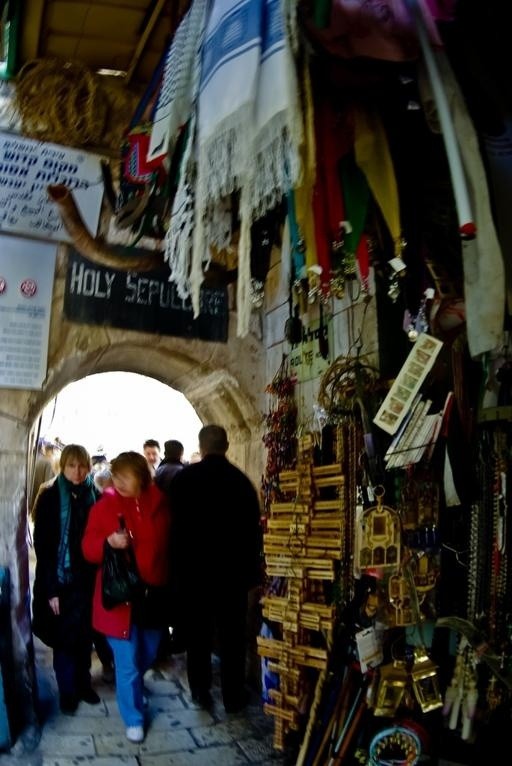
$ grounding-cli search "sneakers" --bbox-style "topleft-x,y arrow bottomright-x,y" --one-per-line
125,724 -> 147,744
142,636 -> 187,698
53,665 -> 116,716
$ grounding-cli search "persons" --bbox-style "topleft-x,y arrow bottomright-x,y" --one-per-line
27,419 -> 268,746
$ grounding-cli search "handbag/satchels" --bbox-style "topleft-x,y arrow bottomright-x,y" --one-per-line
99,514 -> 152,613
